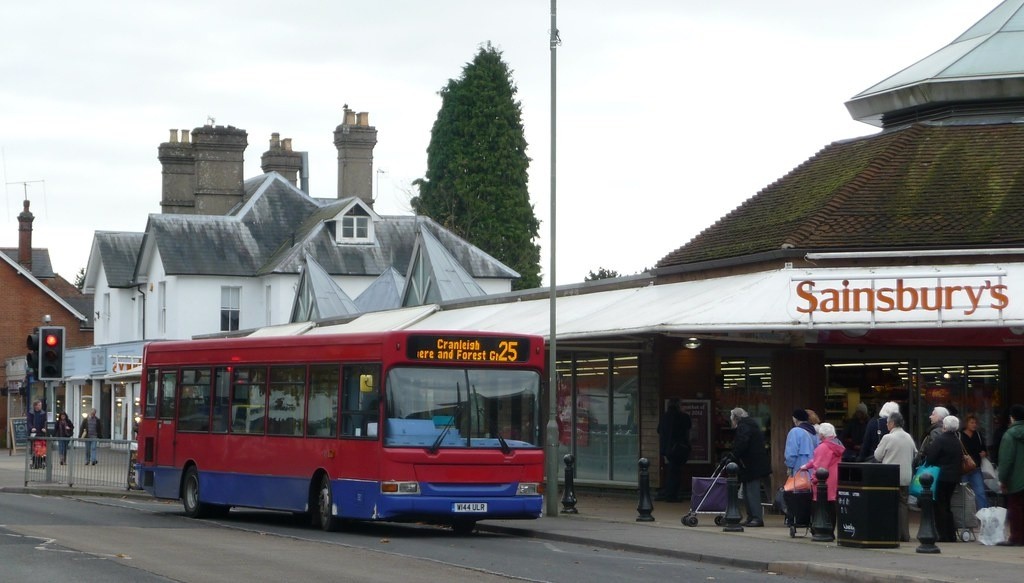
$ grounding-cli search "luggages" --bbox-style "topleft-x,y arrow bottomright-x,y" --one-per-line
783,468 -> 814,538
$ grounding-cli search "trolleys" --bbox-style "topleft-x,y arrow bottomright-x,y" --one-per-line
681,451 -> 730,528
953,476 -> 979,542
781,469 -> 813,537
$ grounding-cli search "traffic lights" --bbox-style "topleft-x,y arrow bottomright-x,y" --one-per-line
39,325 -> 65,380
26,334 -> 39,380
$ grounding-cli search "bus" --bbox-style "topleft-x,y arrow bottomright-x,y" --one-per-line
133,334 -> 545,535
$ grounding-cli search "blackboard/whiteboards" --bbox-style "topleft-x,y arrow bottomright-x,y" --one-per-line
10,417 -> 31,450
126,442 -> 144,490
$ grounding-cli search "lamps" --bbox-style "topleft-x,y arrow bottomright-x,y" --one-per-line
681,337 -> 703,350
941,368 -> 951,379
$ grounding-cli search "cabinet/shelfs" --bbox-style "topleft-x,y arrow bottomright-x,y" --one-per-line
824,393 -> 848,431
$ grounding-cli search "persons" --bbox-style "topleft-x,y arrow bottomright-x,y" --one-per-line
53,413 -> 74,465
784,401 -> 1024,546
27,401 -> 47,468
729,407 -> 767,527
78,409 -> 101,465
658,398 -> 692,500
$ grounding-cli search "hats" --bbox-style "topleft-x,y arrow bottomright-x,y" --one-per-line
792,408 -> 809,421
1010,404 -> 1024,417
732,408 -> 748,417
857,404 -> 867,414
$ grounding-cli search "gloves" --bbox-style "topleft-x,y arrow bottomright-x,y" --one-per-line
720,452 -> 736,466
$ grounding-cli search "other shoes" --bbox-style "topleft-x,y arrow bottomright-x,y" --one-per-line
669,496 -> 683,503
995,541 -> 1024,546
741,519 -> 764,527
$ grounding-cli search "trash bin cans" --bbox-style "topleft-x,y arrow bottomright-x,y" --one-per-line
836,462 -> 901,549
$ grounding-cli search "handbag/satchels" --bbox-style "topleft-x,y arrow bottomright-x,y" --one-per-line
980,457 -> 996,480
784,469 -> 811,491
909,461 -> 940,500
950,485 -> 979,528
864,454 -> 882,463
773,486 -> 789,516
959,439 -> 976,472
65,429 -> 73,437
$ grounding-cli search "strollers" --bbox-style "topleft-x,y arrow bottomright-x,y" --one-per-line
29,428 -> 47,469
